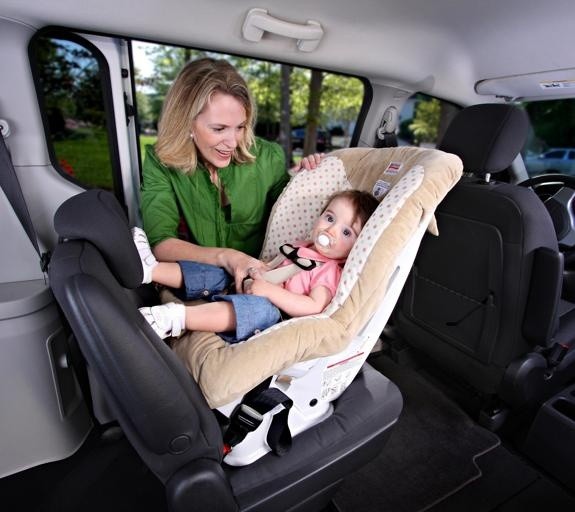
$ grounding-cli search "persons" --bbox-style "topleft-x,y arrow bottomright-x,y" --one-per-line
136,57 -> 325,294
130,188 -> 380,344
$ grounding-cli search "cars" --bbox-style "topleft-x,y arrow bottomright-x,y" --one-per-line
291,130 -> 329,153
526,148 -> 574,175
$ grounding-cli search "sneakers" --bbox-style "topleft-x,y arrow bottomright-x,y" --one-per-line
137,301 -> 184,339
132,225 -> 159,285
